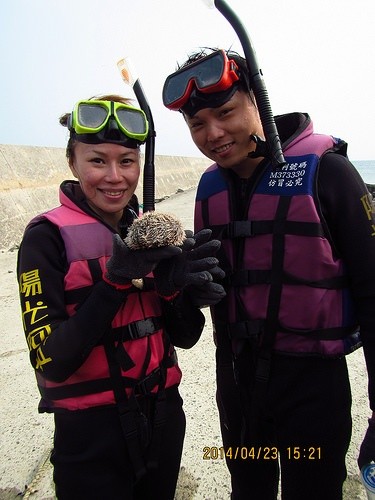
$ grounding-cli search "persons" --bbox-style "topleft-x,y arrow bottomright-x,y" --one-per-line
161,45 -> 375,500
15,92 -> 221,500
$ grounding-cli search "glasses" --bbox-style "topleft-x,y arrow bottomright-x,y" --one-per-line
66,99 -> 150,140
163,50 -> 239,110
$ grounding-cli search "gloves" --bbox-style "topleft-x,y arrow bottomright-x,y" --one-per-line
151,237 -> 221,295
182,228 -> 226,306
103,234 -> 181,284
356,417 -> 375,471
